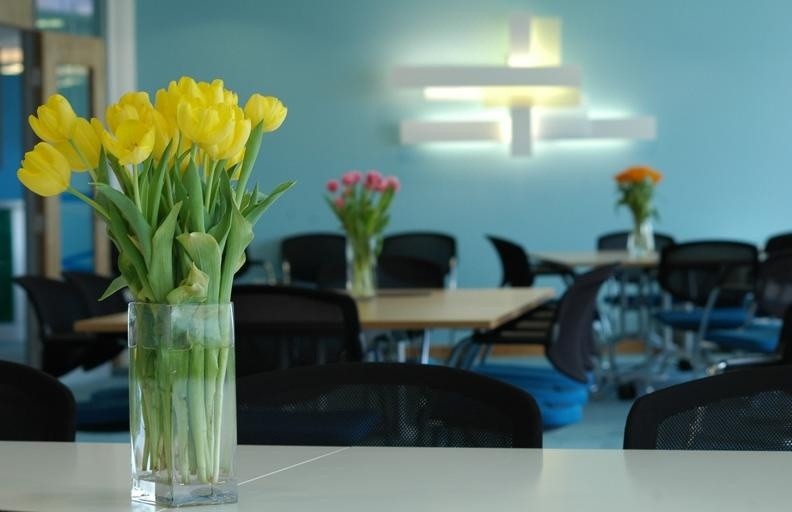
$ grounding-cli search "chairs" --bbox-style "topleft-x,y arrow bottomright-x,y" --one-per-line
622,365 -> 791,453
227,281 -> 365,445
16,262 -> 132,376
655,232 -> 789,364
275,232 -> 350,288
596,232 -> 676,252
0,361 -> 78,442
484,233 -> 574,292
307,364 -> 547,447
471,260 -> 622,424
375,232 -> 457,292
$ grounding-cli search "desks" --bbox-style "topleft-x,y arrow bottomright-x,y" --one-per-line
73,287 -> 553,334
1,442 -> 792,512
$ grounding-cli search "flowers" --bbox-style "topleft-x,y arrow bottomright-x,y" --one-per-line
15,72 -> 299,305
322,170 -> 399,237
614,166 -> 661,218
